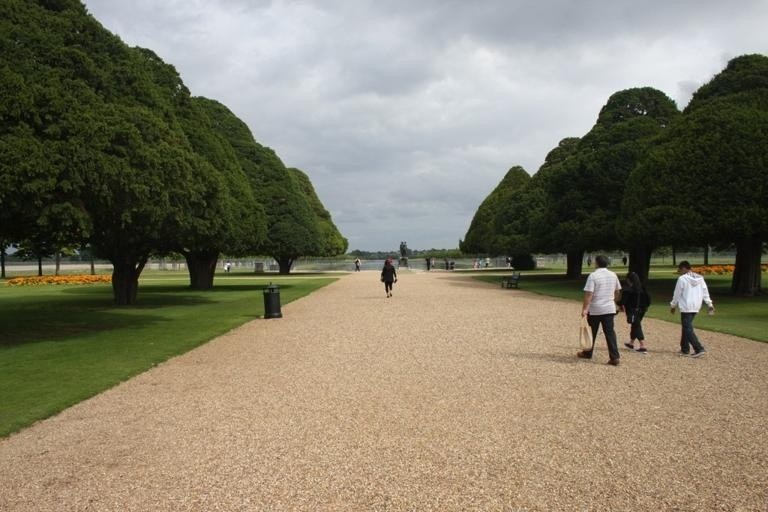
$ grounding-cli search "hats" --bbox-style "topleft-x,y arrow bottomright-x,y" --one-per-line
385,259 -> 391,263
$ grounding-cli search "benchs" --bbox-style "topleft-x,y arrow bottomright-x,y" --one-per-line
502,271 -> 520,289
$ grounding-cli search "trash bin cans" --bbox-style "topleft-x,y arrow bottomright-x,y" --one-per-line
263,281 -> 282,318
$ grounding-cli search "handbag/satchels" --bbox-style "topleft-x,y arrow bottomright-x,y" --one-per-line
627,314 -> 634,323
579,326 -> 593,350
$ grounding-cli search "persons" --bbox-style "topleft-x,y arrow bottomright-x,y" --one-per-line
622,255 -> 627,265
380,254 -> 398,298
424,255 -> 436,273
577,255 -> 623,365
472,254 -> 490,269
353,257 -> 362,272
584,255 -> 592,268
400,240 -> 408,257
503,255 -> 513,267
607,256 -> 612,267
500,270 -> 519,290
223,260 -> 232,272
444,256 -> 455,273
670,260 -> 715,359
617,271 -> 651,352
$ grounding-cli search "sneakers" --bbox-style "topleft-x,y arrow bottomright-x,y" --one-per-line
675,349 -> 706,357
387,293 -> 392,298
577,351 -> 593,358
625,342 -> 647,354
606,358 -> 619,365
